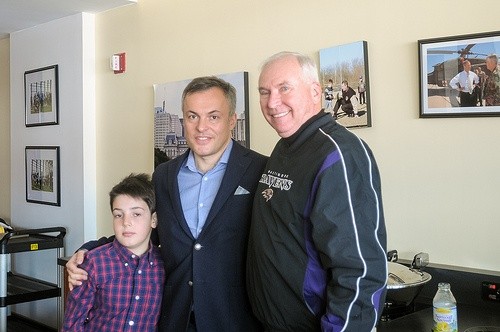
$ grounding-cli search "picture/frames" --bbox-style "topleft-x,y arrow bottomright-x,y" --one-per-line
24,145 -> 61,207
418,30 -> 500,118
24,64 -> 59,127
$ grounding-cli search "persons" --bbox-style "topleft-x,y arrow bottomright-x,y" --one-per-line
449,55 -> 500,107
35,173 -> 43,190
246,51 -> 389,332
61,172 -> 165,332
34,90 -> 44,107
324,75 -> 366,120
66,76 -> 270,332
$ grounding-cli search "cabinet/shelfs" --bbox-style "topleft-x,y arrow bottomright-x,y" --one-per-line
0,227 -> 66,332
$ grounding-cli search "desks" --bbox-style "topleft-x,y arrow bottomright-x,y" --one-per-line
377,302 -> 500,332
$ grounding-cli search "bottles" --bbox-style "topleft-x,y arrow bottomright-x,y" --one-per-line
433,283 -> 458,332
